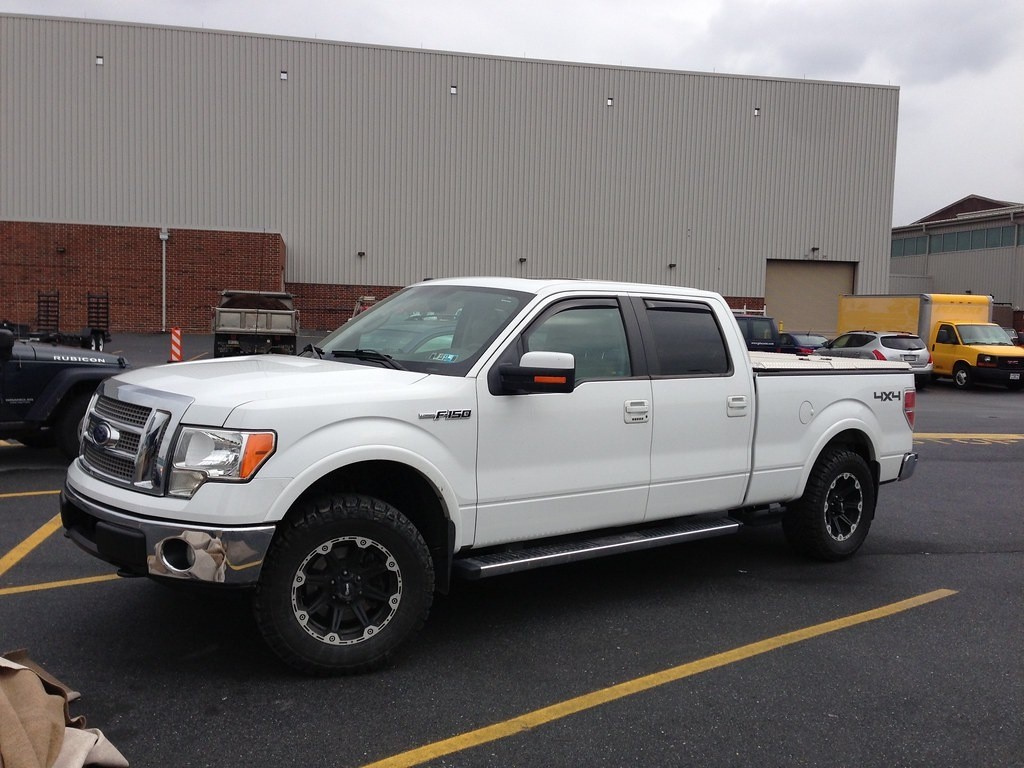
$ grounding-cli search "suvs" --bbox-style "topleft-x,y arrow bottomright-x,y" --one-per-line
813,329 -> 933,393
0,322 -> 128,455
733,313 -> 783,354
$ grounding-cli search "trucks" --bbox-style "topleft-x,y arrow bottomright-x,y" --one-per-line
836,293 -> 1024,391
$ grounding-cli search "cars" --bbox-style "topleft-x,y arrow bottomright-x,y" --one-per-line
779,331 -> 829,358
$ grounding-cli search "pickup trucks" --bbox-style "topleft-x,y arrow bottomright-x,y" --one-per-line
212,288 -> 300,357
62,276 -> 920,678
